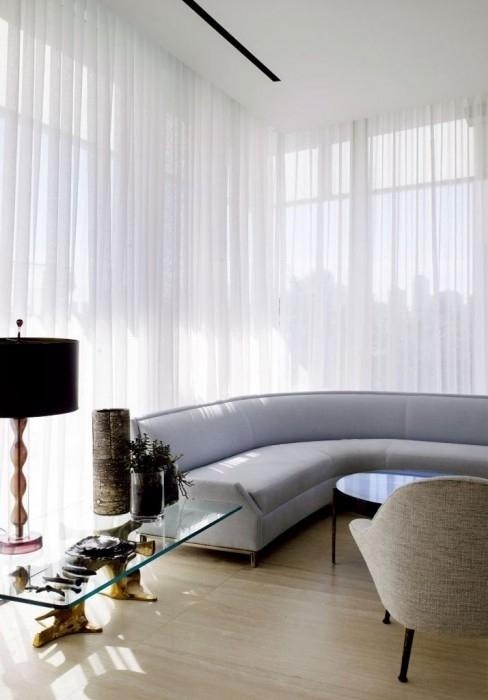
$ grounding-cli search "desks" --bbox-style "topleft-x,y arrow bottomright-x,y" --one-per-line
0,498 -> 243,647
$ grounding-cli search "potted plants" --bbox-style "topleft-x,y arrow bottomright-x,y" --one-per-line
106,433 -> 195,524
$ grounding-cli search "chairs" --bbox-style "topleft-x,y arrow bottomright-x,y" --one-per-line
348,475 -> 488,683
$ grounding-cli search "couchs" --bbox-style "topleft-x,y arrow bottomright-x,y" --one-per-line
130,391 -> 488,568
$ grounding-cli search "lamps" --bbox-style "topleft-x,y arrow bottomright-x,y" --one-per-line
0,319 -> 79,554
